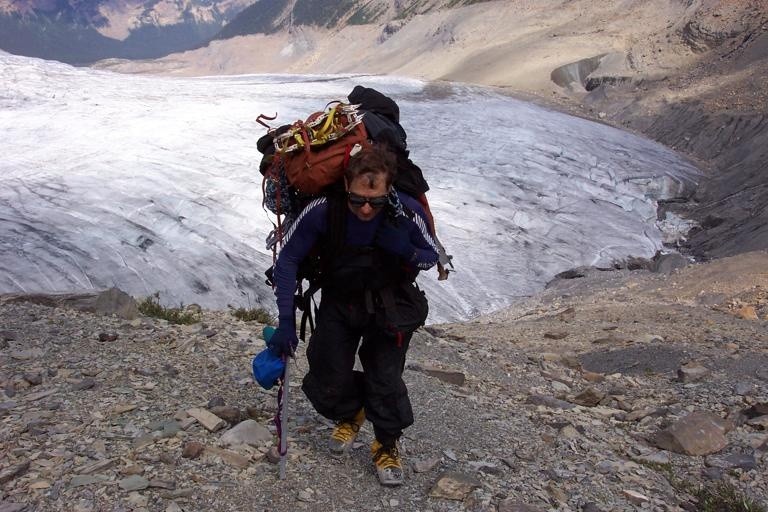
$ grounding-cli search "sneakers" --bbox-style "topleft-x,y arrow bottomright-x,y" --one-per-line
327,404 -> 365,460
374,449 -> 404,486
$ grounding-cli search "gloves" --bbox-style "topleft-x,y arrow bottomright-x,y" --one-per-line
376,225 -> 417,262
270,315 -> 299,359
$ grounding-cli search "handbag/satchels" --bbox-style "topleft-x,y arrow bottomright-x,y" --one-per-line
255,101 -> 405,218
369,281 -> 430,331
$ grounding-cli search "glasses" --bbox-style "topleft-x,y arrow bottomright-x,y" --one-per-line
347,190 -> 389,210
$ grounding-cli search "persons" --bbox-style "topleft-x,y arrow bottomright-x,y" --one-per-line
259,137 -> 441,491
